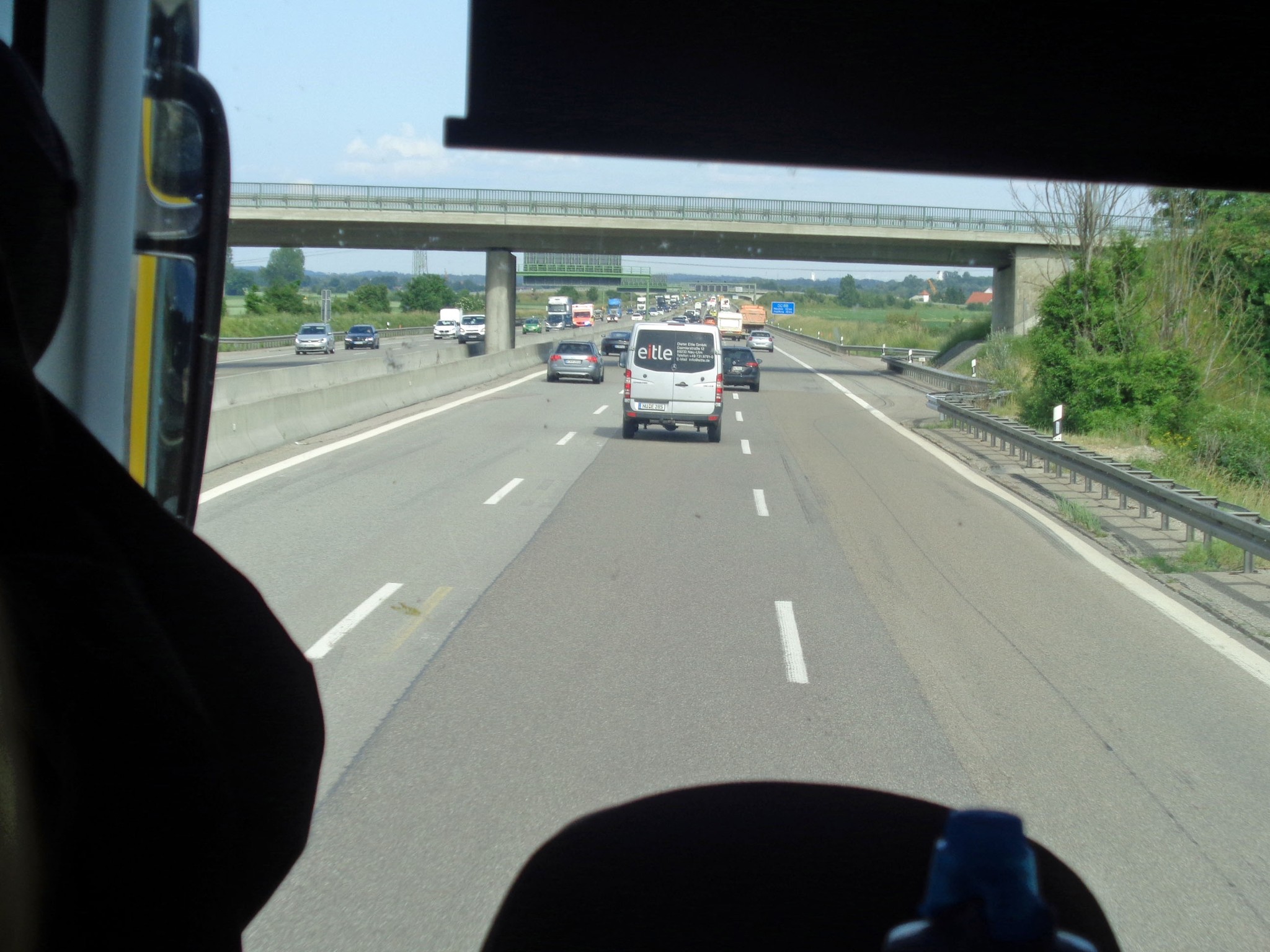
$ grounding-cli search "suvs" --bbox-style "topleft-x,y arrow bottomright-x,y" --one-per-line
593,309 -> 604,321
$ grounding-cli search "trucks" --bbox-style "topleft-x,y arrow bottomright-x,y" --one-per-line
717,312 -> 743,341
432,308 -> 463,340
457,314 -> 486,344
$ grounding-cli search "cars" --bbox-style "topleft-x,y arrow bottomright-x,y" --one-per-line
521,318 -> 542,334
625,292 -> 731,325
721,346 -> 763,392
600,330 -> 632,356
607,313 -> 618,323
744,330 -> 774,353
544,314 -> 565,332
618,321 -> 725,443
547,340 -> 605,384
344,324 -> 380,350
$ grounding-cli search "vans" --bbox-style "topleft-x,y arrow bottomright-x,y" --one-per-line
294,322 -> 335,355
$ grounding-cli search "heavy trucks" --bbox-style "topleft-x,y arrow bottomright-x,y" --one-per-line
571,303 -> 594,327
608,297 -> 622,319
545,295 -> 573,317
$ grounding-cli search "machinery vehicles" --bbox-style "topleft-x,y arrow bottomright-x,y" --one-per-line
736,305 -> 768,330
